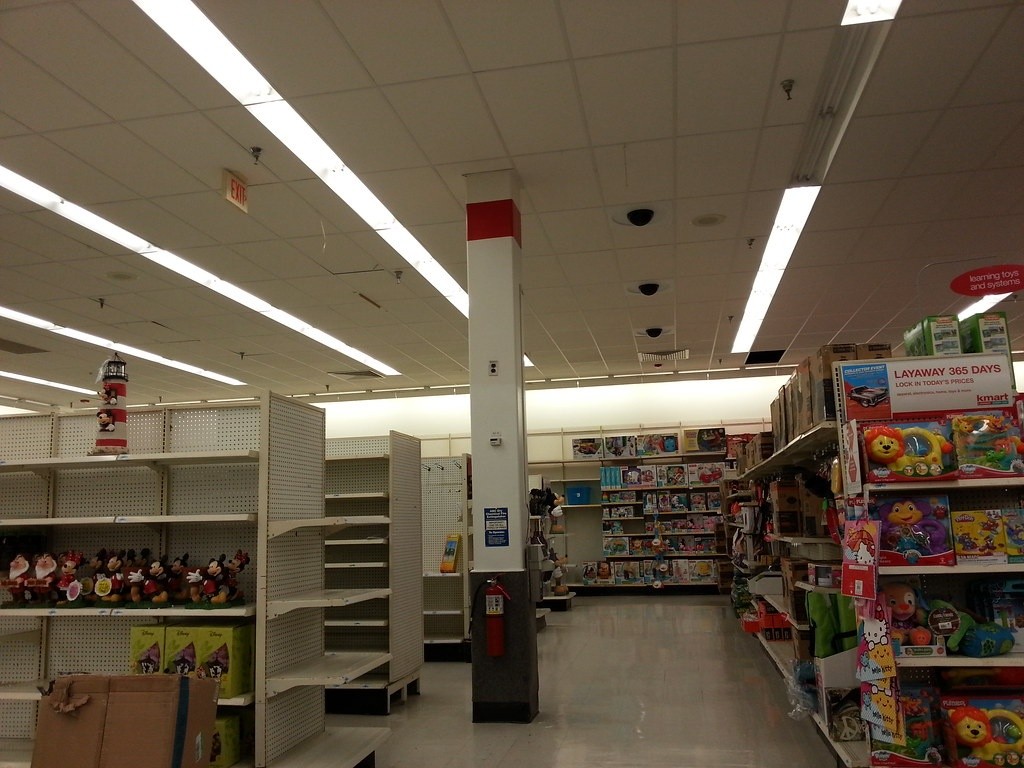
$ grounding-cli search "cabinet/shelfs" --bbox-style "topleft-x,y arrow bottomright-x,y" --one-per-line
725,312 -> 1024,768
0,390 -> 575,768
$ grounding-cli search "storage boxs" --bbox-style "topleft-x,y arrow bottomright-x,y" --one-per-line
31,625 -> 248,768
440,534 -> 462,573
528,452 -> 724,587
727,312 -> 1023,768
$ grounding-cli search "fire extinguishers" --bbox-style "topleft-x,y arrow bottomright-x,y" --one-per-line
485,574 -> 510,658
